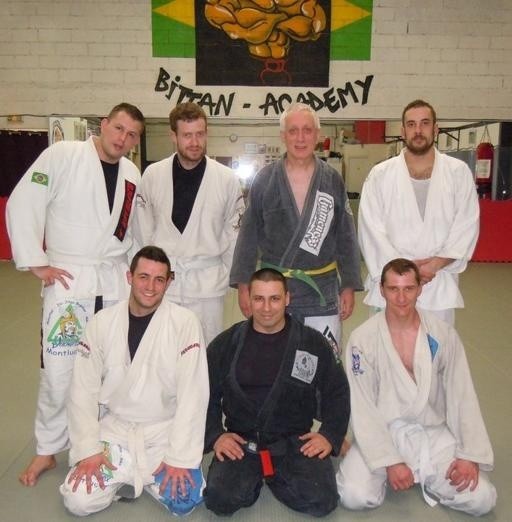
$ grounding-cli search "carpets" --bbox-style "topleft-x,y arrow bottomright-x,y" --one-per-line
467,199 -> 511,264
0,196 -> 46,260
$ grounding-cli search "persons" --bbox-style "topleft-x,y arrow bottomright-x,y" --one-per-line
358,100 -> 481,327
203,269 -> 352,517
229,102 -> 364,360
128,102 -> 246,345
6,103 -> 146,486
58,246 -> 211,518
335,258 -> 497,517
